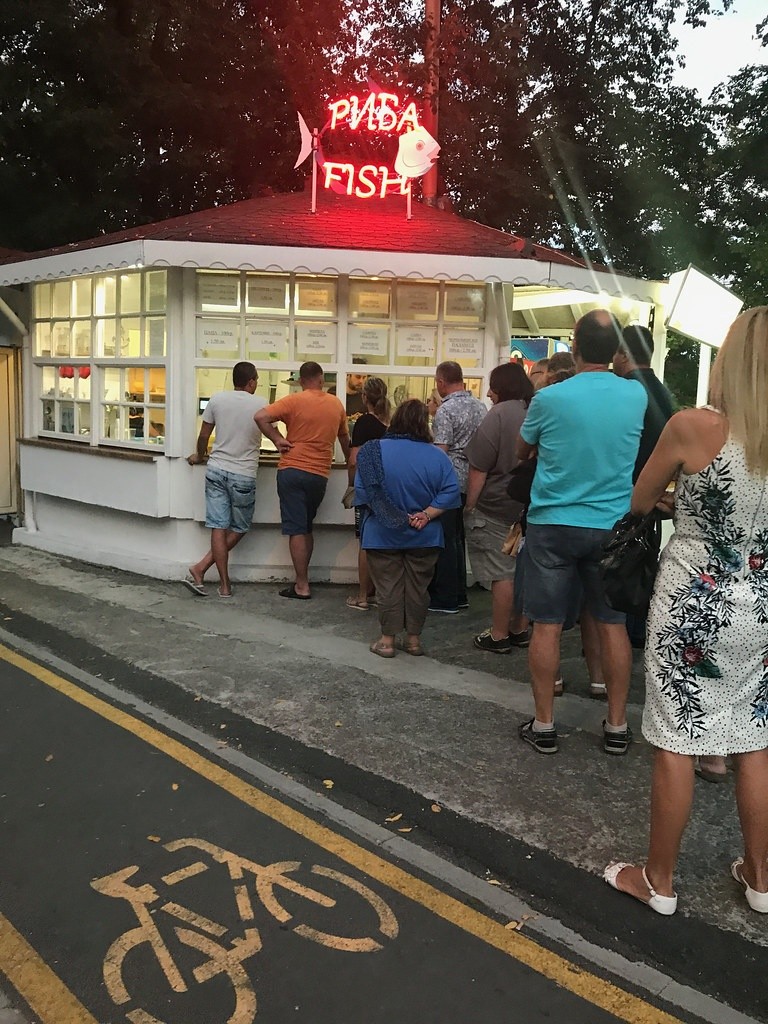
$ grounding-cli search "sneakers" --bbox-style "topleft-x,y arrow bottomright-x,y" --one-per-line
429,604 -> 459,614
600,718 -> 633,754
459,595 -> 471,608
590,683 -> 608,701
472,630 -> 509,653
552,678 -> 563,695
507,629 -> 530,648
520,716 -> 560,752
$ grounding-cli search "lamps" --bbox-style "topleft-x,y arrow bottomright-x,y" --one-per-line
663,264 -> 744,349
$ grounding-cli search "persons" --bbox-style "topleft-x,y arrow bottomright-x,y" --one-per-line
515,306 -> 648,757
526,350 -> 580,400
178,361 -> 284,597
602,299 -> 768,919
342,379 -> 387,610
352,399 -> 462,660
425,361 -> 489,615
107,394 -> 162,440
585,325 -> 675,647
462,361 -> 539,654
428,388 -> 445,440
327,358 -> 371,424
253,360 -> 351,599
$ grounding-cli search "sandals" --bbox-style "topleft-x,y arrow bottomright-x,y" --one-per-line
691,755 -> 728,782
604,859 -> 677,916
397,639 -> 423,655
366,597 -> 379,606
345,596 -> 368,611
369,640 -> 395,658
732,856 -> 768,914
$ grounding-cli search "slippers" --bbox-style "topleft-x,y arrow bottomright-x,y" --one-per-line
278,583 -> 313,600
216,583 -> 233,598
183,580 -> 209,595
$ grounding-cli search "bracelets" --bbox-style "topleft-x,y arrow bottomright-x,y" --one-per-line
422,510 -> 432,522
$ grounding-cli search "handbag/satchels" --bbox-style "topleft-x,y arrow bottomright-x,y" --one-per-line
500,521 -> 527,556
598,504 -> 662,614
